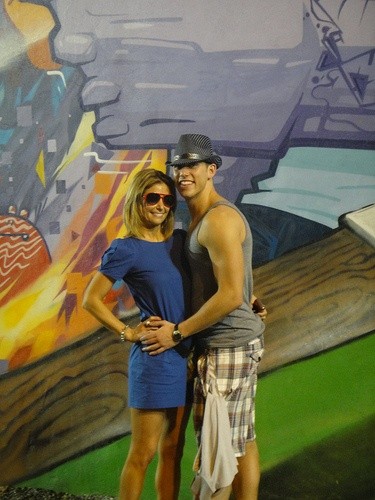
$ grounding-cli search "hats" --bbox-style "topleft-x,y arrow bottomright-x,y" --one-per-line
166,134 -> 223,169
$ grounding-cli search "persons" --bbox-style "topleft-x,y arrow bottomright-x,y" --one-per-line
140,133 -> 265,500
83,168 -> 267,500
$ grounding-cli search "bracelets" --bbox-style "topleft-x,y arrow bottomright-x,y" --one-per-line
121,324 -> 129,341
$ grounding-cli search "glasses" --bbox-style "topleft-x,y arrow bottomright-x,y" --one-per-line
142,193 -> 177,208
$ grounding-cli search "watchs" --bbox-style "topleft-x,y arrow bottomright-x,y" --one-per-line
172,323 -> 183,342
